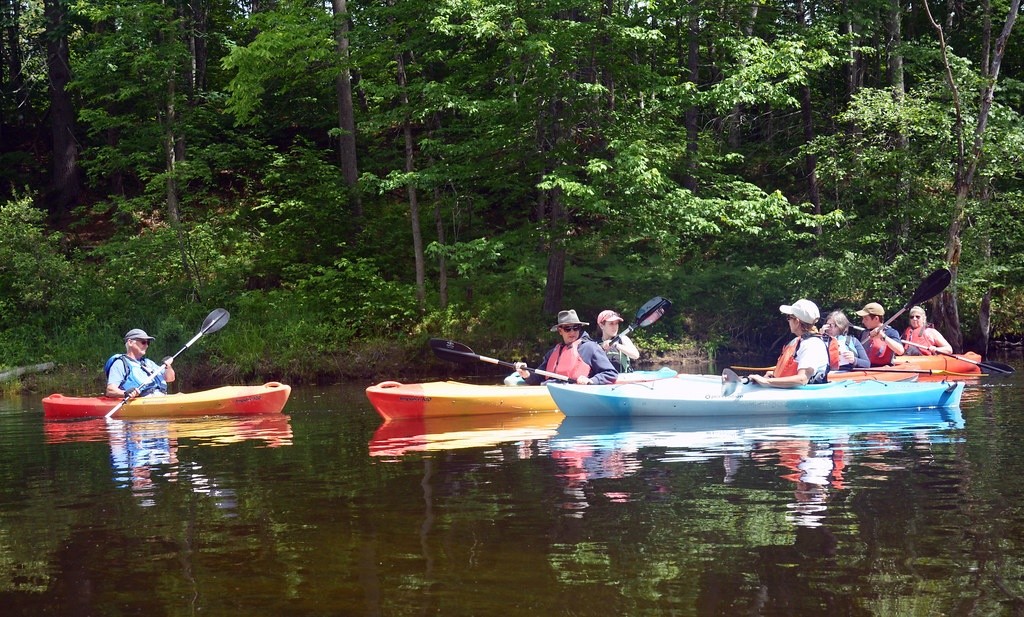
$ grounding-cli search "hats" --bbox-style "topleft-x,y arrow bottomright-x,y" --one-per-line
125,329 -> 155,344
597,310 -> 624,325
550,309 -> 590,333
856,302 -> 884,317
779,299 -> 820,325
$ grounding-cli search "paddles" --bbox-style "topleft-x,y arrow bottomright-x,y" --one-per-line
720,367 -> 756,398
430,337 -> 589,385
104,308 -> 231,418
602,294 -> 673,351
860,266 -> 954,345
729,311 -> 1017,376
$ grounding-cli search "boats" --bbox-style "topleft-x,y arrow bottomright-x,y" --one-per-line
896,351 -> 984,375
42,408 -> 296,448
545,373 -> 966,415
40,381 -> 292,418
365,409 -> 568,462
554,403 -> 968,454
364,366 -> 678,422
824,366 -> 922,383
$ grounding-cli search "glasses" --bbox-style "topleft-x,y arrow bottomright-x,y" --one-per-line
131,339 -> 150,346
910,315 -> 923,320
786,315 -> 796,321
826,323 -> 838,328
559,325 -> 581,332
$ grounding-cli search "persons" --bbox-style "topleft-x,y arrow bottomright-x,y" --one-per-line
855,303 -> 905,366
105,329 -> 175,398
901,306 -> 953,356
597,310 -> 640,373
820,311 -> 871,372
749,299 -> 831,389
514,310 -> 618,385
